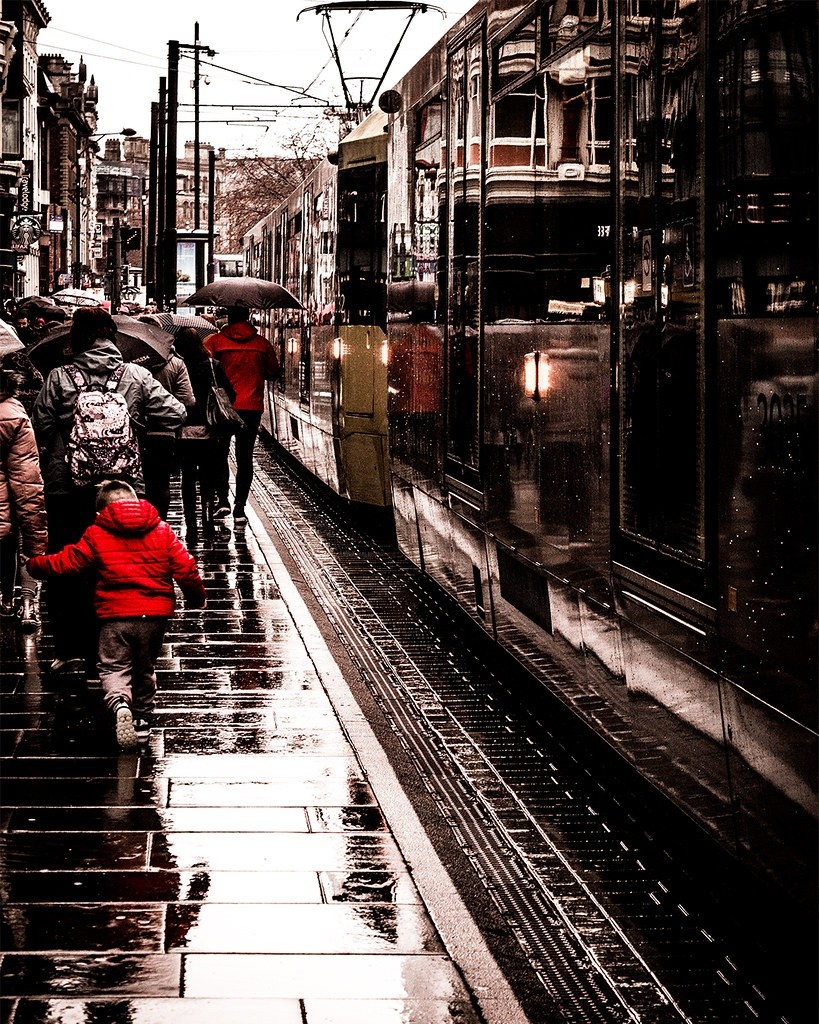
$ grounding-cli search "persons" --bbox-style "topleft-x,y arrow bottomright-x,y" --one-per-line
7,311 -> 42,352
0,352 -> 49,620
165,327 -> 237,546
201,305 -> 282,524
22,478 -> 207,751
17,551 -> 44,629
0,286 -> 74,332
131,334 -> 196,521
31,304 -> 187,671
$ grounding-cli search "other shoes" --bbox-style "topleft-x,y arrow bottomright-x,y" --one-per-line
51,649 -> 82,670
186,527 -> 199,540
131,719 -> 153,743
213,502 -> 231,518
202,503 -> 212,521
114,702 -> 138,749
0,595 -> 15,616
233,511 -> 247,523
21,599 -> 42,628
87,672 -> 103,692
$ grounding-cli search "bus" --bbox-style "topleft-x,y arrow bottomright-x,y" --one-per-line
246,1 -> 819,870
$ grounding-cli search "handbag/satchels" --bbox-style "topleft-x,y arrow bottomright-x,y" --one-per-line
206,358 -> 246,434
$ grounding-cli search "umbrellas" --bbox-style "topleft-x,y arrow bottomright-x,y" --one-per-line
0,288 -> 219,368
181,276 -> 308,310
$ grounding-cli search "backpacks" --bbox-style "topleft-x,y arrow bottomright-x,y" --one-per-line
68,362 -> 139,487
